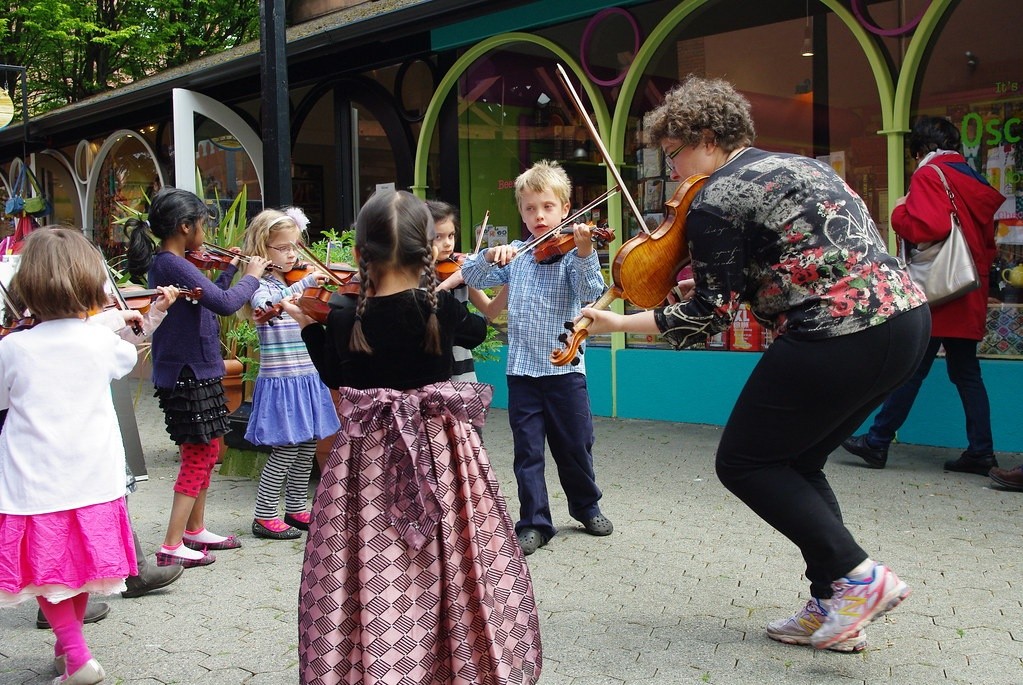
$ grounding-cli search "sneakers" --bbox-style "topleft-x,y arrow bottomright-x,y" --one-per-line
809,560 -> 912,650
766,596 -> 867,652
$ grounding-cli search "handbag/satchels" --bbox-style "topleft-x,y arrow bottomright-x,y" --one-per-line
897,163 -> 982,308
5,165 -> 53,220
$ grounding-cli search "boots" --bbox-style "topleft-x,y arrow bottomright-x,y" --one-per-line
36,601 -> 110,629
119,531 -> 184,598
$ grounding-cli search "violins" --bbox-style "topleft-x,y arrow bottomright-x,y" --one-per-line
252,280 -> 360,328
549,169 -> 712,368
434,251 -> 473,290
102,285 -> 207,317
285,259 -> 358,287
0,315 -> 40,341
531,221 -> 615,263
185,244 -> 274,274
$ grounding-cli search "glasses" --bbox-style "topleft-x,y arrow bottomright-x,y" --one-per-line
265,244 -> 295,254
664,139 -> 690,170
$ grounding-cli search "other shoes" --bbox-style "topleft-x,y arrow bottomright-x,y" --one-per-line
251,518 -> 302,540
155,545 -> 217,569
52,658 -> 105,685
284,512 -> 310,532
182,533 -> 242,551
55,654 -> 66,675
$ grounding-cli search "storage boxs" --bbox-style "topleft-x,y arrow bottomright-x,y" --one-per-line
707,303 -> 766,350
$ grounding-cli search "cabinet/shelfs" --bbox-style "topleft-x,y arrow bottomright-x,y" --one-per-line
519,113 -> 666,266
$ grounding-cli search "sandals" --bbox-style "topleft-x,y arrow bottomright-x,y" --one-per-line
569,509 -> 614,536
515,528 -> 548,556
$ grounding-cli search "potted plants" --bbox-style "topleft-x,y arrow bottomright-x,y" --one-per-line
111,169 -> 503,474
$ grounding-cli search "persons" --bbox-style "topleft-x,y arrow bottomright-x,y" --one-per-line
572,77 -> 931,652
123,185 -> 271,569
0,228 -> 135,685
245,207 -> 341,539
279,188 -> 543,685
461,160 -> 613,558
6,274 -> 183,629
418,200 -> 509,383
840,114 -> 1007,476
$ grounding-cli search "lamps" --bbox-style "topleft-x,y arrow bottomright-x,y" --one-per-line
573,85 -> 587,157
801,0 -> 814,56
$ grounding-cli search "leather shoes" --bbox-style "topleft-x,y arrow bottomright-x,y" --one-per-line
987,464 -> 1023,492
943,449 -> 999,477
841,434 -> 887,469
